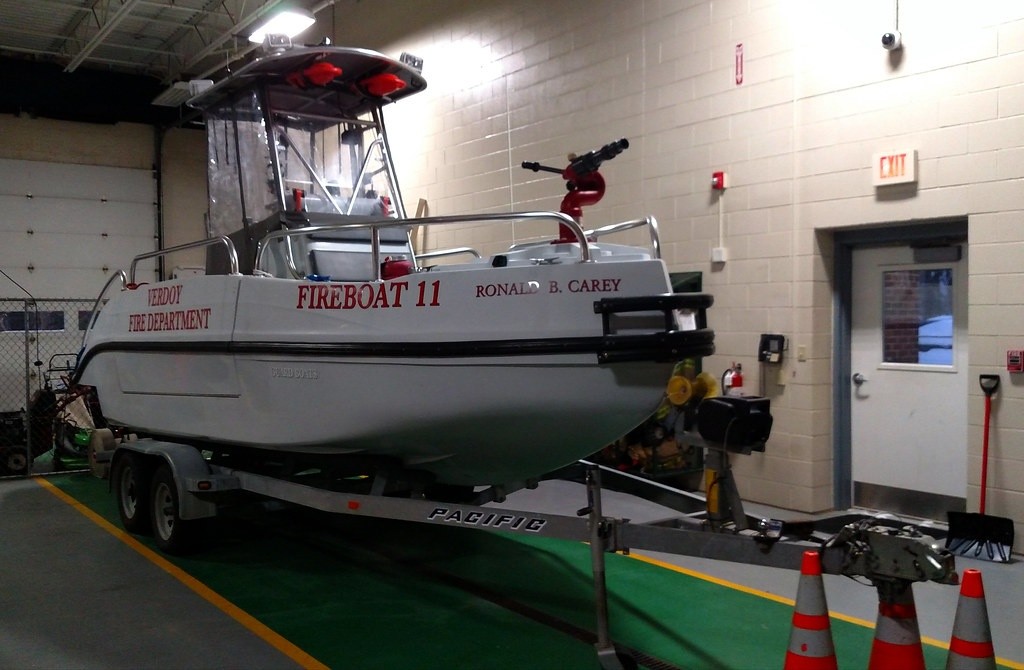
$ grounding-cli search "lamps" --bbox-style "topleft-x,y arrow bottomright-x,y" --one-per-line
246,1 -> 318,44
151,81 -> 188,107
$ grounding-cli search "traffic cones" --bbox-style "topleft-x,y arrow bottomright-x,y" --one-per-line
867,583 -> 927,669
784,550 -> 838,670
946,569 -> 998,670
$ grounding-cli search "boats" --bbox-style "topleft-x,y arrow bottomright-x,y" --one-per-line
70,37 -> 716,497
